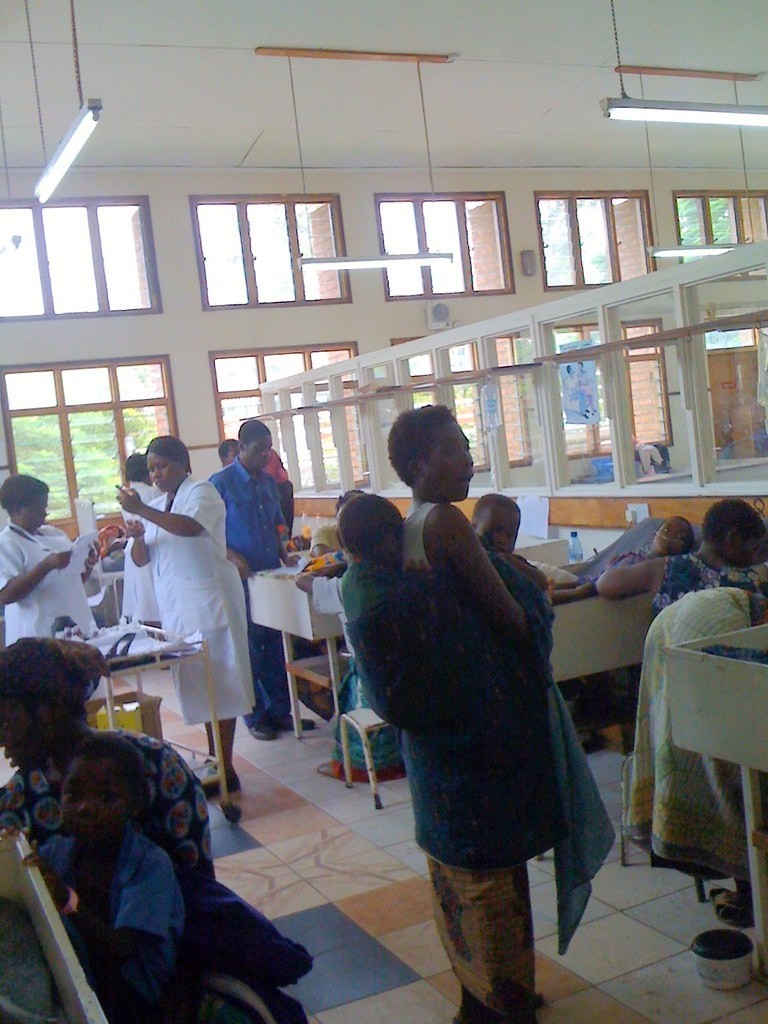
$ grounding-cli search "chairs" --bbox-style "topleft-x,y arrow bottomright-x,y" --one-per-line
309,613 -> 390,811
73,497 -> 127,623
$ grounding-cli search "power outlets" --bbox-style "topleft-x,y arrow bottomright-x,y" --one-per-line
626,502 -> 650,522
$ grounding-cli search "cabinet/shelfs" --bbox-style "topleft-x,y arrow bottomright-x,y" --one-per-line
73,625 -> 242,823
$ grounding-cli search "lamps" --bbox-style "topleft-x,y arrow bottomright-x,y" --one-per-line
255,47 -> 453,272
599,0 -> 768,127
25,0 -> 103,203
615,66 -> 766,258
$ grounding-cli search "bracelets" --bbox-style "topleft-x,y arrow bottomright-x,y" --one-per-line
60,886 -> 78,915
85,558 -> 94,570
38,561 -> 49,575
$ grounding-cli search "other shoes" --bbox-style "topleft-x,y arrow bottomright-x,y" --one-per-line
203,775 -> 241,799
276,715 -> 315,730
249,721 -> 278,740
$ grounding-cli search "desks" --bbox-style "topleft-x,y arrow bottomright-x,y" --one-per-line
248,562 -> 347,738
664,624 -> 768,985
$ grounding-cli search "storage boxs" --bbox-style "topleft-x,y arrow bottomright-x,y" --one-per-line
95,703 -> 140,736
84,692 -> 164,741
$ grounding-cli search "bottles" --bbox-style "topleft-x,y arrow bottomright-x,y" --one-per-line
567,531 -> 583,565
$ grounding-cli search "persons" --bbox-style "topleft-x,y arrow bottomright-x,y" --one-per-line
116,436 -> 256,799
206,420 -> 316,739
0,474 -> 101,647
551,516 -> 694,606
120,454 -> 172,624
295,403 -> 554,1024
596,499 -> 768,641
0,638 -> 216,1024
